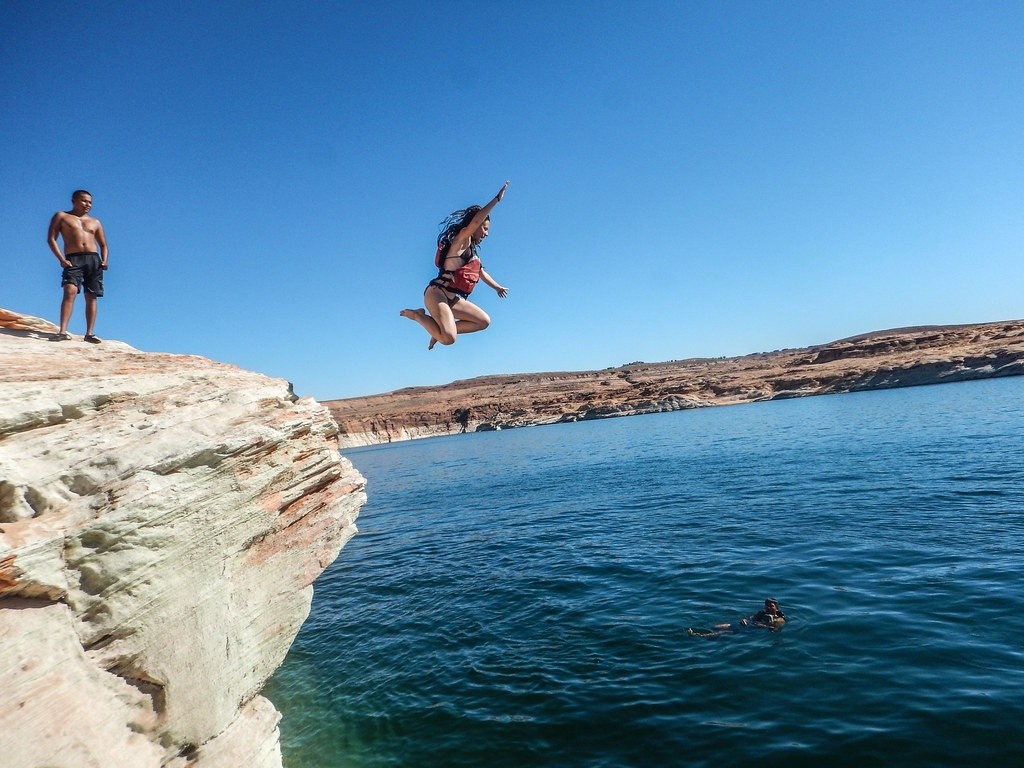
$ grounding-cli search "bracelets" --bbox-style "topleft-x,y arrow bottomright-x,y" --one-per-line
496,194 -> 500,201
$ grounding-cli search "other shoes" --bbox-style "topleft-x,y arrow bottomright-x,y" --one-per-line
84,334 -> 102,344
49,334 -> 72,342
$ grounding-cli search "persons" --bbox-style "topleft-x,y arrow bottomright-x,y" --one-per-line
689,597 -> 786,637
400,181 -> 511,350
47,190 -> 108,342
